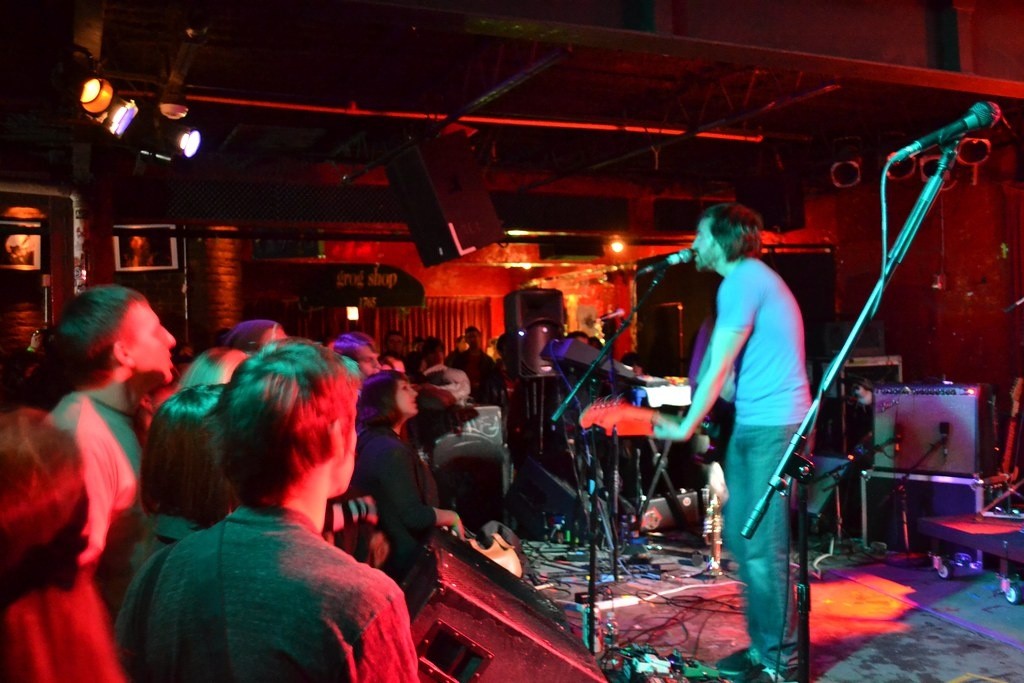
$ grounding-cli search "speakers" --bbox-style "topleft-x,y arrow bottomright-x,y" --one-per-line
503,287 -> 565,378
871,382 -> 992,478
396,527 -> 609,683
652,301 -> 685,375
388,130 -> 508,268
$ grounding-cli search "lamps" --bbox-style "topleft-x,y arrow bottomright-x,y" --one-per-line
914,128 -> 954,182
50,59 -> 115,116
877,130 -> 916,182
80,94 -> 139,140
145,117 -> 203,160
945,111 -> 992,167
830,135 -> 869,189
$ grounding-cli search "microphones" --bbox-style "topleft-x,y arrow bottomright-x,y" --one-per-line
939,421 -> 951,457
893,423 -> 903,453
638,249 -> 693,274
888,101 -> 996,165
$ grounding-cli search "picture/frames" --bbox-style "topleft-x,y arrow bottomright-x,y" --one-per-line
112,222 -> 185,275
0,222 -> 41,270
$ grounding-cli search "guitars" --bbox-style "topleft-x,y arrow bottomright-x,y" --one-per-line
580,393 -> 737,468
984,376 -> 1024,516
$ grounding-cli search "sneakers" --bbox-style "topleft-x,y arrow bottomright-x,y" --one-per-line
717,647 -> 798,683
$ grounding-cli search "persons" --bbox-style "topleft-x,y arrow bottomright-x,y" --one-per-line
652,202 -> 816,683
0,285 -> 644,683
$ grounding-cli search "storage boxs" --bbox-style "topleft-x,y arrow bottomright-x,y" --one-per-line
860,470 -> 985,561
